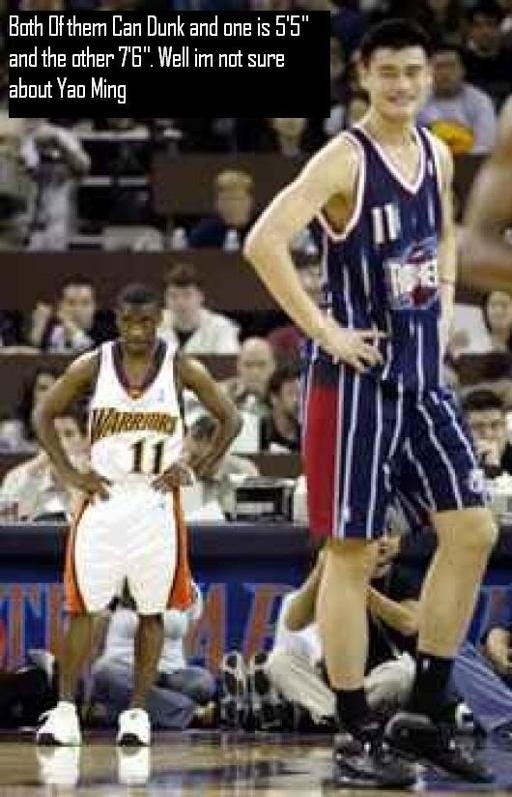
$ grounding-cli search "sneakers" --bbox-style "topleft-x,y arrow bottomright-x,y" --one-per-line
116,747 -> 151,785
116,708 -> 151,745
334,713 -> 494,787
197,701 -> 216,725
36,707 -> 81,745
36,746 -> 79,783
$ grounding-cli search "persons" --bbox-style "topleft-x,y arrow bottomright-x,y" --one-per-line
450,581 -> 512,749
30,284 -> 242,745
264,507 -> 420,726
83,574 -> 220,729
0,1 -> 512,526
34,744 -> 151,795
242,17 -> 500,789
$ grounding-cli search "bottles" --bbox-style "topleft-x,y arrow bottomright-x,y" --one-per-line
52,327 -> 65,352
223,229 -> 240,250
290,230 -> 317,256
2,493 -> 19,522
293,475 -> 308,524
172,227 -> 188,249
244,393 -> 258,417
